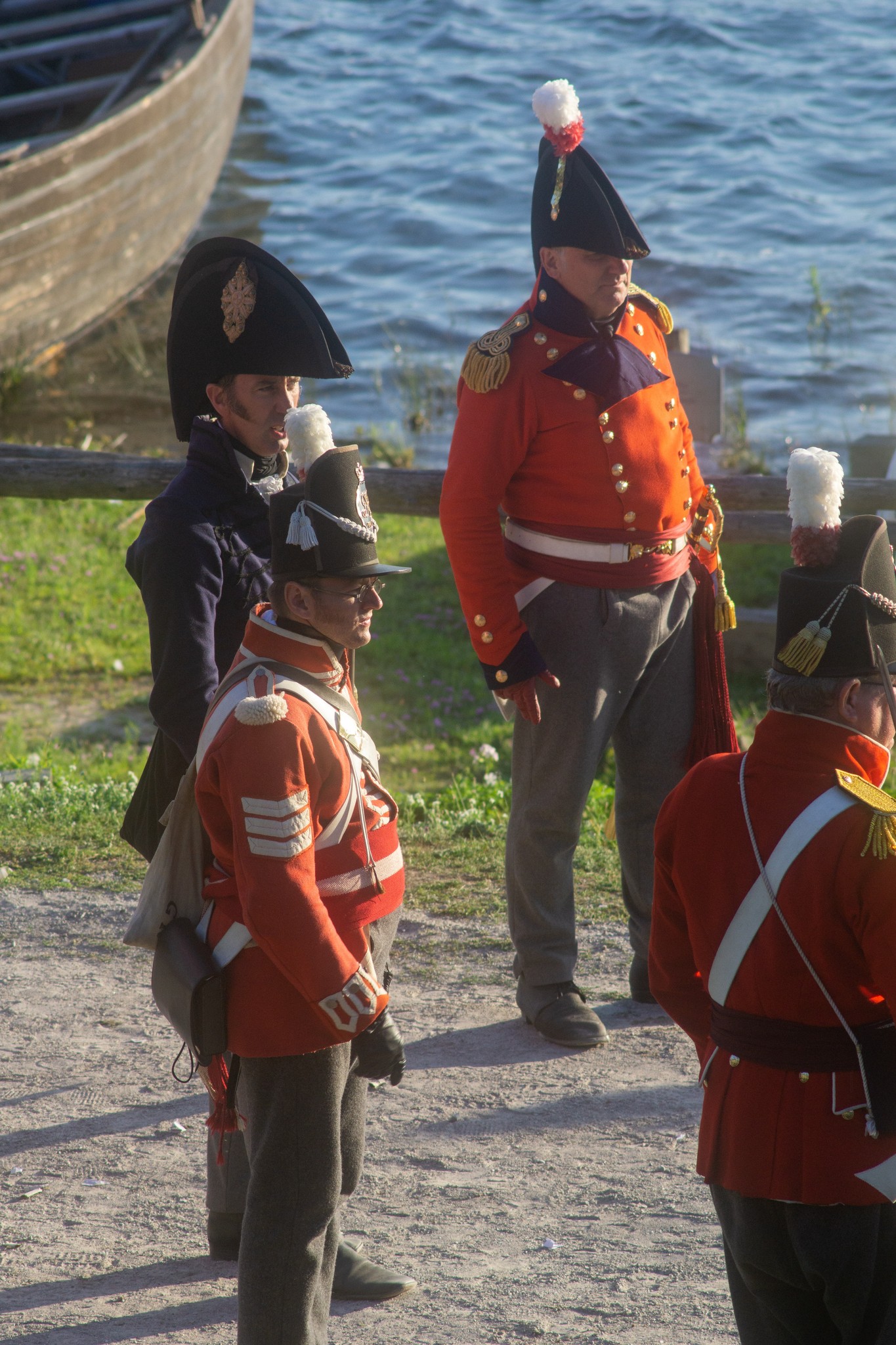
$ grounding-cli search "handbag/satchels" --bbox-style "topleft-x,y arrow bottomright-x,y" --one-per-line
122,755 -> 212,949
119,731 -> 214,871
151,917 -> 228,1067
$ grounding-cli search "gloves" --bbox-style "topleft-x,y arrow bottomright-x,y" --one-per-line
354,1008 -> 406,1086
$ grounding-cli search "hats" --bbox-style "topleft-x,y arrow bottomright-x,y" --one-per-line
268,444 -> 414,580
165,237 -> 355,441
530,78 -> 650,278
770,446 -> 895,681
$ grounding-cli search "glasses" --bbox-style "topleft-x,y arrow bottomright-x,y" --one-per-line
298,580 -> 385,602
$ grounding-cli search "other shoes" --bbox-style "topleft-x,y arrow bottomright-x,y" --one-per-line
629,955 -> 656,1002
515,973 -> 608,1046
331,1238 -> 417,1298
206,1213 -> 245,1260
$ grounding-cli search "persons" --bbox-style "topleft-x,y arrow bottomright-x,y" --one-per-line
652,449 -> 896,1345
192,402 -> 406,1345
439,79 -> 734,1043
119,239 -> 410,1302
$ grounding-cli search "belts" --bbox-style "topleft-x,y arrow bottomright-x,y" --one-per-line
503,516 -> 688,566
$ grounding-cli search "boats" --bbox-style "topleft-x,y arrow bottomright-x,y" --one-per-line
0,0 -> 255,389
